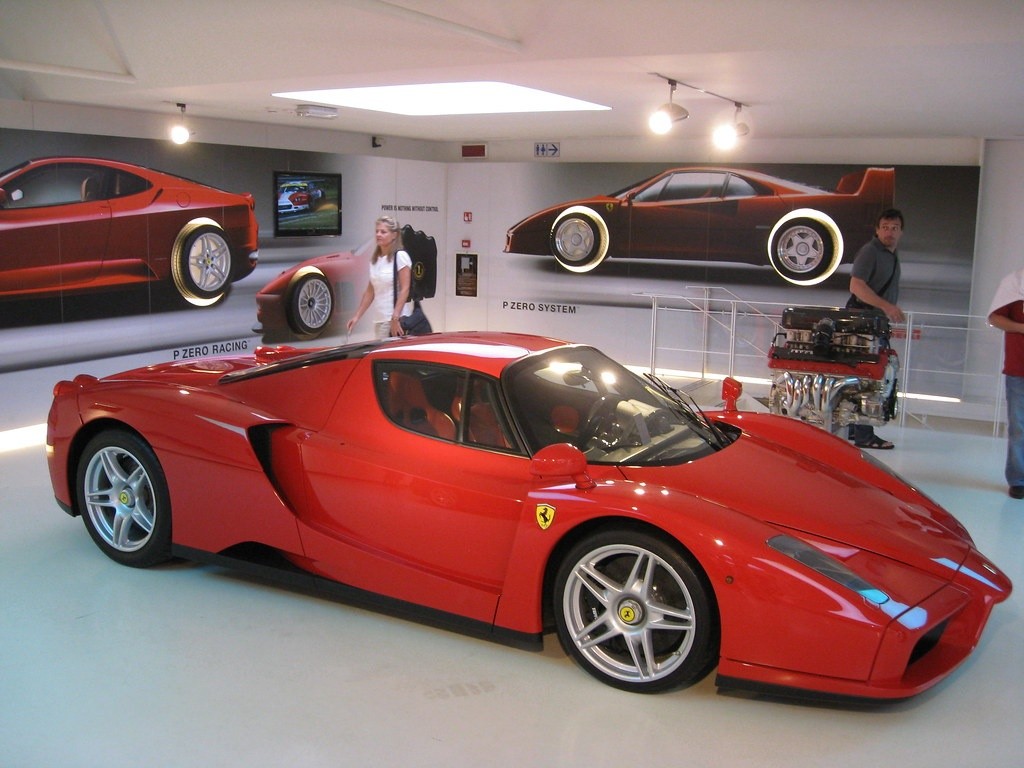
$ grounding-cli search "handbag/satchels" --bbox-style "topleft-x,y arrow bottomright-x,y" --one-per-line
389,307 -> 432,337
846,295 -> 865,310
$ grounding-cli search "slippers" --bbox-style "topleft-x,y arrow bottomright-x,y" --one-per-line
855,436 -> 894,449
848,434 -> 855,440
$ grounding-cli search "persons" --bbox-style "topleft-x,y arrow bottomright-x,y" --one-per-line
844,210 -> 905,449
985,269 -> 1024,499
346,215 -> 414,340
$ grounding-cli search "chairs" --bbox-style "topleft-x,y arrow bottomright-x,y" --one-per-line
384,372 -> 458,442
448,375 -> 512,449
79,176 -> 97,200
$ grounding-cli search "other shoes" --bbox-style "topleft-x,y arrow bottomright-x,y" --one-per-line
1009,484 -> 1024,499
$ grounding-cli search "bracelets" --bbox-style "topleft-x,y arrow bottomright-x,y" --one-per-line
391,316 -> 400,321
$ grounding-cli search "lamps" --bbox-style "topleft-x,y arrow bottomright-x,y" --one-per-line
712,103 -> 751,148
171,104 -> 197,144
651,80 -> 689,133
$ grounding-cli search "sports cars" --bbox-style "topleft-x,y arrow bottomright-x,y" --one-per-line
46,331 -> 1014,701
0,155 -> 259,310
254,238 -> 377,335
503,167 -> 894,285
278,182 -> 322,214
